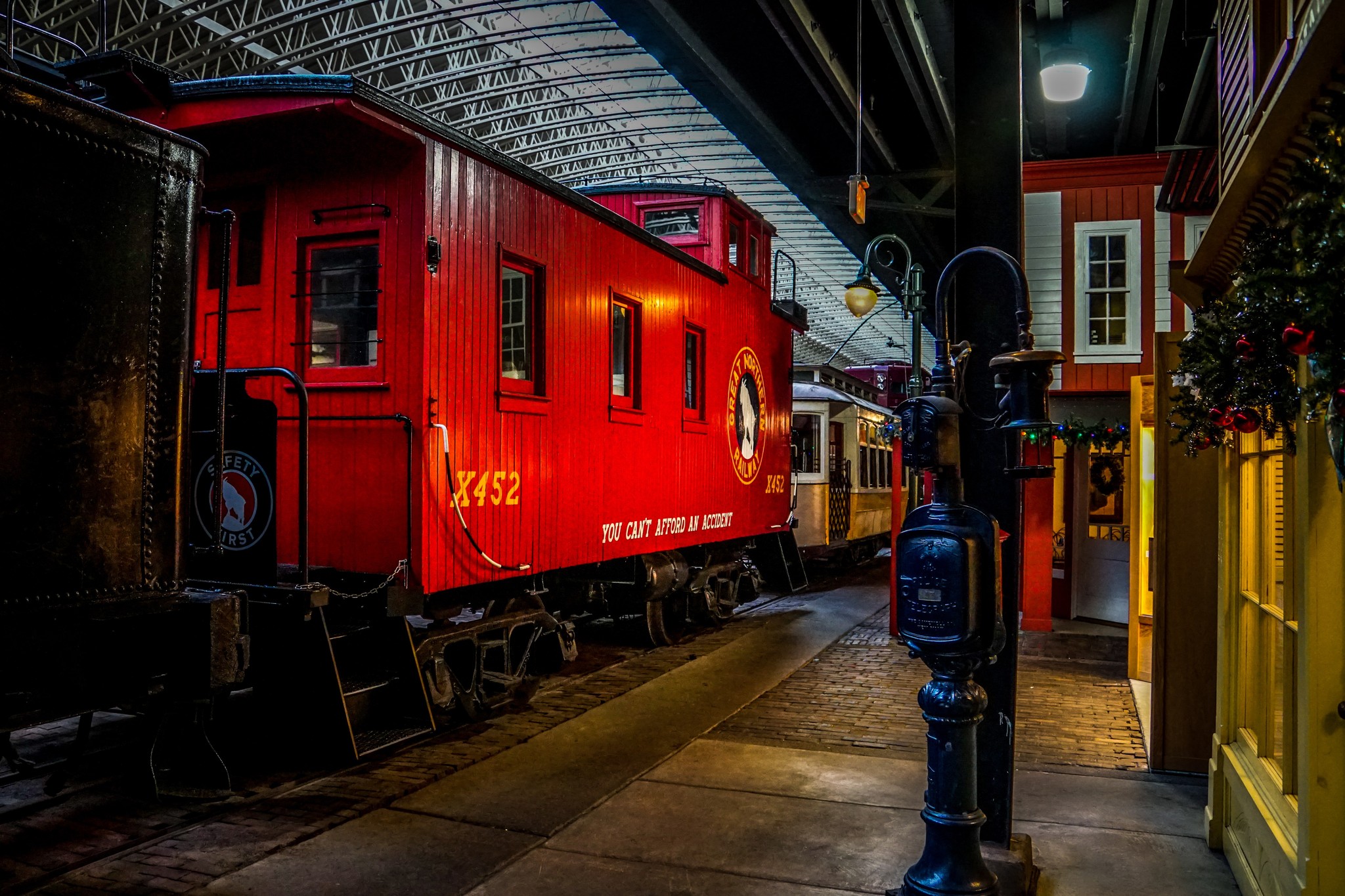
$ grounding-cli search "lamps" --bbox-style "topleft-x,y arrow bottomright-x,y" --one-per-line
1037,48 -> 1097,106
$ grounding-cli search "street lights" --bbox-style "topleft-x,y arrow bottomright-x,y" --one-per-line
844,235 -> 927,517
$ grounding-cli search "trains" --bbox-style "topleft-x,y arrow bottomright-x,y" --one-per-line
1,45 -> 919,806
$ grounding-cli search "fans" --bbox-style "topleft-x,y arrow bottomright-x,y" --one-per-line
880,337 -> 907,350
